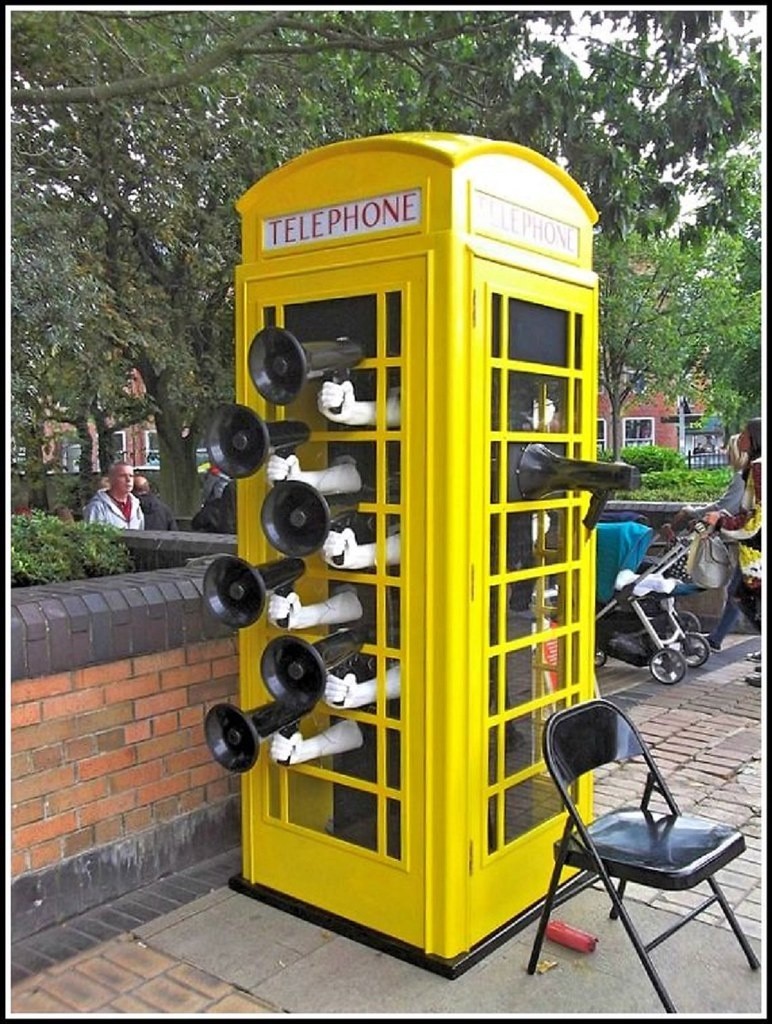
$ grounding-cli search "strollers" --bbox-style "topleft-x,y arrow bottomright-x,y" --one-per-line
596,509 -> 731,685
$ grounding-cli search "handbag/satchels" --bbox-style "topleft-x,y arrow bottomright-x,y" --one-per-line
690,537 -> 731,590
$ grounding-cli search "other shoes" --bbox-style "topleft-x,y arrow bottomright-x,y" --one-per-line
756,666 -> 761,672
749,676 -> 761,688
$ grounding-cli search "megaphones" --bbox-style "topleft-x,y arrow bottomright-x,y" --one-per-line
205,699 -> 301,773
248,325 -> 362,415
260,627 -> 365,715
518,443 -> 642,542
259,480 -> 359,565
202,556 -> 305,629
206,403 -> 311,486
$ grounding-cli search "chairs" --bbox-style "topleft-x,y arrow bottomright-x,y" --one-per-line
525,698 -> 760,1013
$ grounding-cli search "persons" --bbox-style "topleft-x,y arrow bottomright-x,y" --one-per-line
704,417 -> 761,688
252,376 -> 400,768
134,476 -> 178,531
83,461 -> 145,530
684,434 -> 762,663
191,481 -> 236,534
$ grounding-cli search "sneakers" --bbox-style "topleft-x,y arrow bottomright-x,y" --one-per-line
746,651 -> 761,662
705,636 -> 721,653
